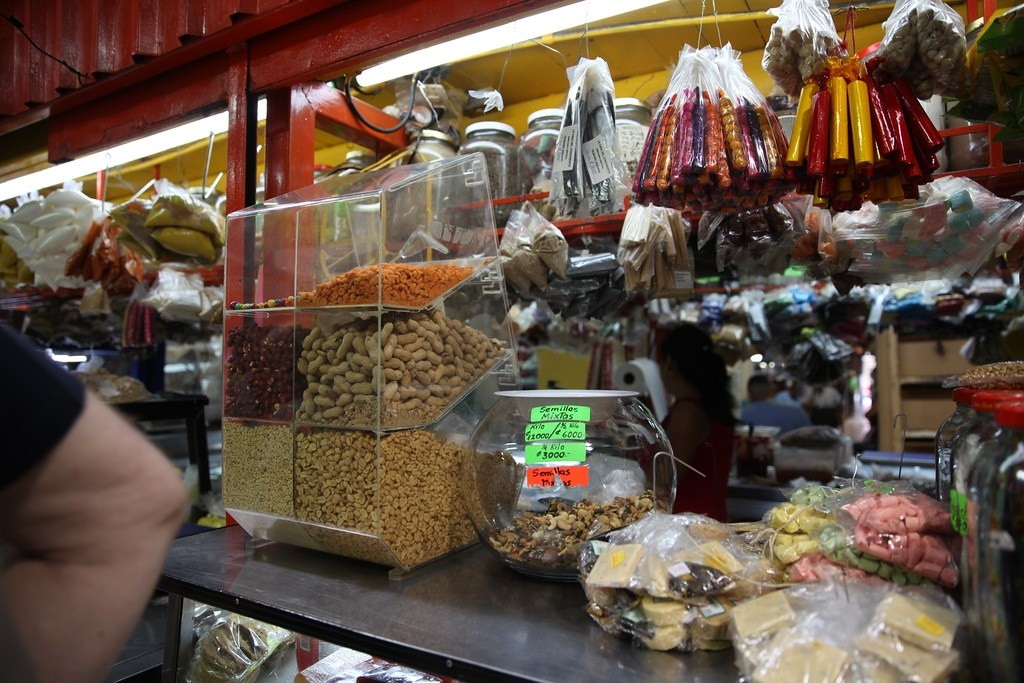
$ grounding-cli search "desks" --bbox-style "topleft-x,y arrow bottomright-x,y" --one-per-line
158,529 -> 750,683
112,389 -> 211,500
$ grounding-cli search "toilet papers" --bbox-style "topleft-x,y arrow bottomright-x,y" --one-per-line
613,359 -> 670,423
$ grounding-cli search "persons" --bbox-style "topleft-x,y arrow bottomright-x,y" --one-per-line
0,322 -> 188,683
650,319 -> 735,523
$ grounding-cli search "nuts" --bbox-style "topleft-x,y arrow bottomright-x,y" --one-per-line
295,430 -> 520,566
874,9 -> 969,101
295,305 -> 508,422
762,25 -> 848,95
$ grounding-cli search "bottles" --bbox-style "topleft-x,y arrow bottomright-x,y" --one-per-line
933,386 -> 1024,683
462,388 -> 678,582
186,96 -> 657,225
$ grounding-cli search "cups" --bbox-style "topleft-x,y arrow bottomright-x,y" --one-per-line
735,425 -> 774,476
